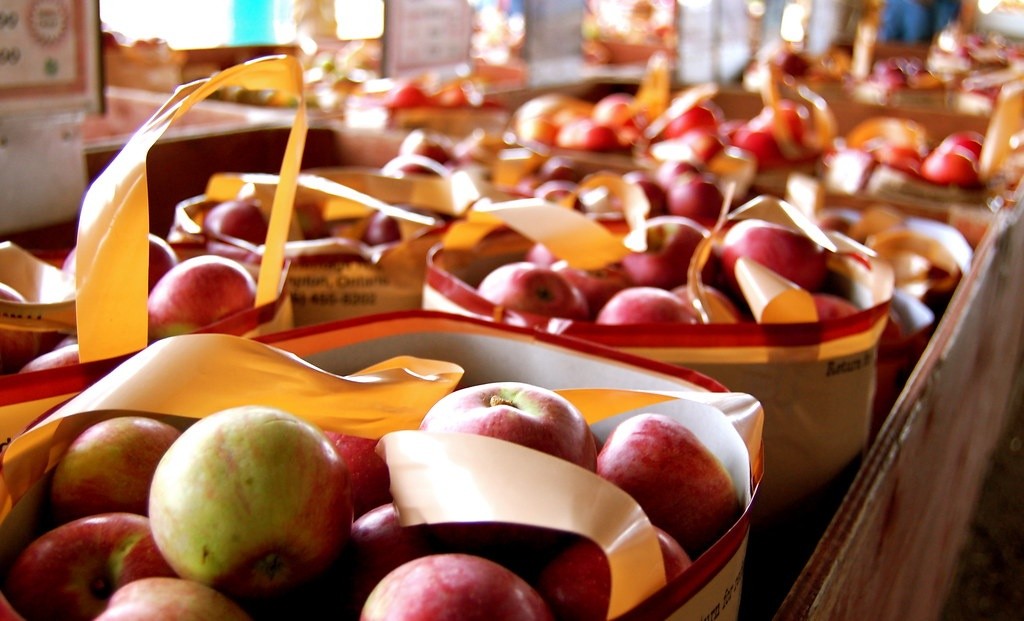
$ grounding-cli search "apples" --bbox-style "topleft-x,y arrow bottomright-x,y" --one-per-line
0,36 -> 1024,621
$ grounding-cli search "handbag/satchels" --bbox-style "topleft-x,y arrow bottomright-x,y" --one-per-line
0,1 -> 1024,621
